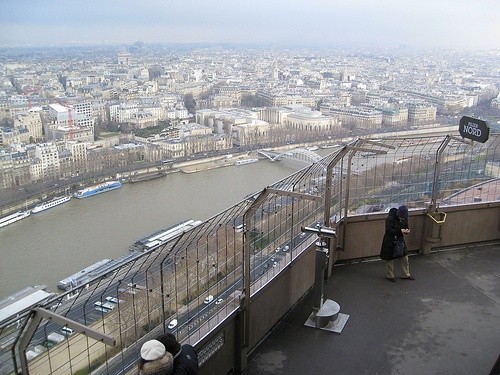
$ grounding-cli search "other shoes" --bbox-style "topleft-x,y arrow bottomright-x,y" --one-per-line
386,277 -> 396,282
402,275 -> 415,280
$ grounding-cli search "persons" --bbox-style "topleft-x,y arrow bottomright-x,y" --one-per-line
151,333 -> 200,375
380,206 -> 415,282
139,339 -> 174,375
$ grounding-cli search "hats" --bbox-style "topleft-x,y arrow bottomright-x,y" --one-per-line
396,206 -> 408,218
140,339 -> 165,361
